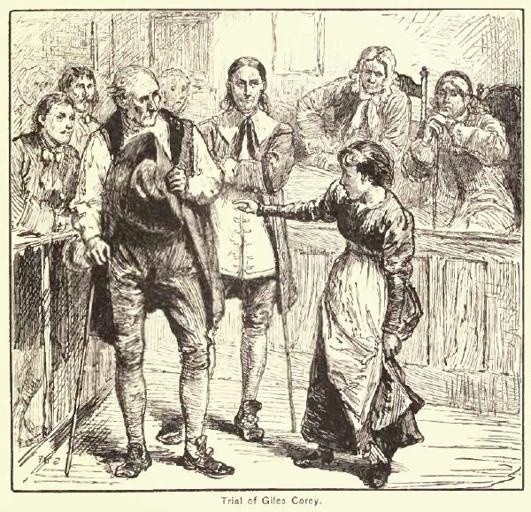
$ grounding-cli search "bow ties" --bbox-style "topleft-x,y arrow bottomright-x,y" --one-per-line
40,149 -> 68,165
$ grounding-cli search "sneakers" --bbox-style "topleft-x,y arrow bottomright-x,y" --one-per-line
295,450 -> 335,468
158,425 -> 186,443
183,437 -> 234,477
370,462 -> 392,487
235,400 -> 265,443
114,441 -> 152,476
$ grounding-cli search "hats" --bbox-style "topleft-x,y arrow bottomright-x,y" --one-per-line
106,133 -> 185,236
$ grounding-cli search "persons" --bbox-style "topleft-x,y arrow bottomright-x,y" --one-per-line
11,45 -> 519,489
